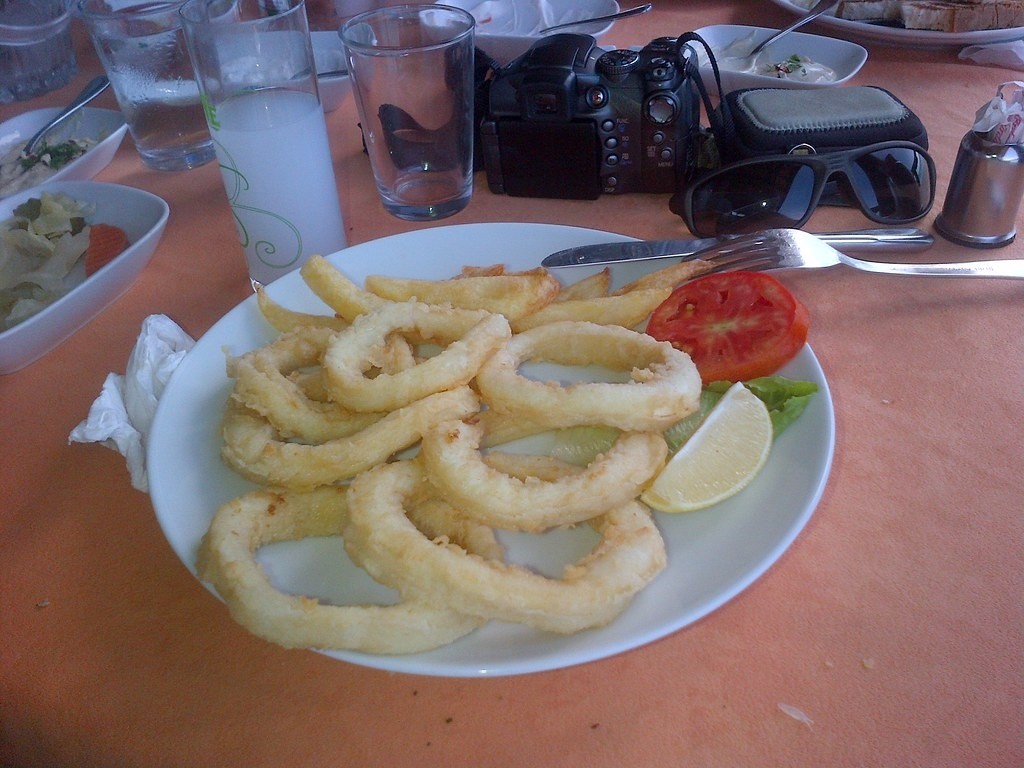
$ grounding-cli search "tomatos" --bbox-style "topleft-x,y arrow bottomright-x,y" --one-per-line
645,272 -> 810,380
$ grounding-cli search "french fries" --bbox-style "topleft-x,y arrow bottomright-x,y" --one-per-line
250,252 -> 716,339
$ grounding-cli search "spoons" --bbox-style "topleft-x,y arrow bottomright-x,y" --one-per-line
532,3 -> 653,35
17,75 -> 110,159
317,67 -> 348,79
718,0 -> 845,73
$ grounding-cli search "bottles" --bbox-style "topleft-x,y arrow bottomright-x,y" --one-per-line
932,130 -> 1024,248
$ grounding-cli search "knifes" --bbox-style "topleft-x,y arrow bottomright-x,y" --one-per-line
539,226 -> 937,273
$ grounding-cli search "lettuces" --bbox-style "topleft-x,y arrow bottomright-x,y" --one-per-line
544,378 -> 817,496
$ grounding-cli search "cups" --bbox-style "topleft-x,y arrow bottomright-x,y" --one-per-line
1,0 -> 76,104
337,3 -> 474,219
64,0 -> 216,172
177,0 -> 353,294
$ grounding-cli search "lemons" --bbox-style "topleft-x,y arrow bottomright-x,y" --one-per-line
639,382 -> 775,514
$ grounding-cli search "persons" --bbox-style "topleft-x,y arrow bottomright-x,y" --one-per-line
878,152 -> 924,219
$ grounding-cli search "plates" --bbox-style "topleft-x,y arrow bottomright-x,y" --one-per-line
775,0 -> 1024,52
143,221 -> 841,678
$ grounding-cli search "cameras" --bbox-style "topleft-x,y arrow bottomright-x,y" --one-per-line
479,33 -> 699,199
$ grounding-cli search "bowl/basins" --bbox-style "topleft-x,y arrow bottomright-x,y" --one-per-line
1,180 -> 171,375
417,0 -> 622,61
683,23 -> 869,99
1,105 -> 131,197
213,28 -> 350,113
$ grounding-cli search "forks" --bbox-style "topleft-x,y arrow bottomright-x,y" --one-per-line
680,228 -> 1024,283
